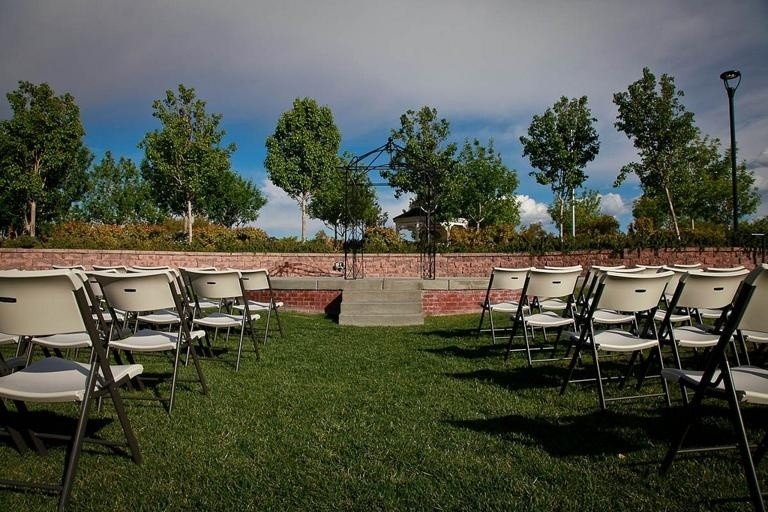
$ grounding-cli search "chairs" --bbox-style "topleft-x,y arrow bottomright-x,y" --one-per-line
477,263 -> 767,512
0,265 -> 284,512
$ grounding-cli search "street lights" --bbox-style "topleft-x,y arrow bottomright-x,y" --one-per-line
721,69 -> 741,232
